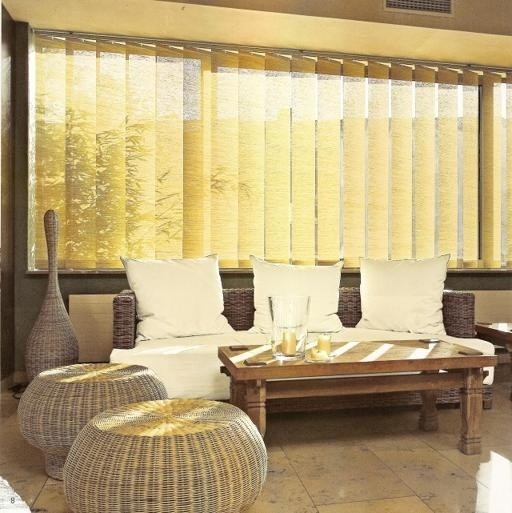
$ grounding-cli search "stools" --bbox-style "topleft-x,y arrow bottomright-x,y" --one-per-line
18,365 -> 267,512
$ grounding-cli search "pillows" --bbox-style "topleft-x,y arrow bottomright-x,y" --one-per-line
353,254 -> 449,337
246,254 -> 345,333
122,253 -> 241,342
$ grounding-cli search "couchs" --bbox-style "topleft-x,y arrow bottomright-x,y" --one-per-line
114,289 -> 491,415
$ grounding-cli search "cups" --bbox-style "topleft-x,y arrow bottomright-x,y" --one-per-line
282,332 -> 296,356
268,295 -> 310,361
317,334 -> 332,357
